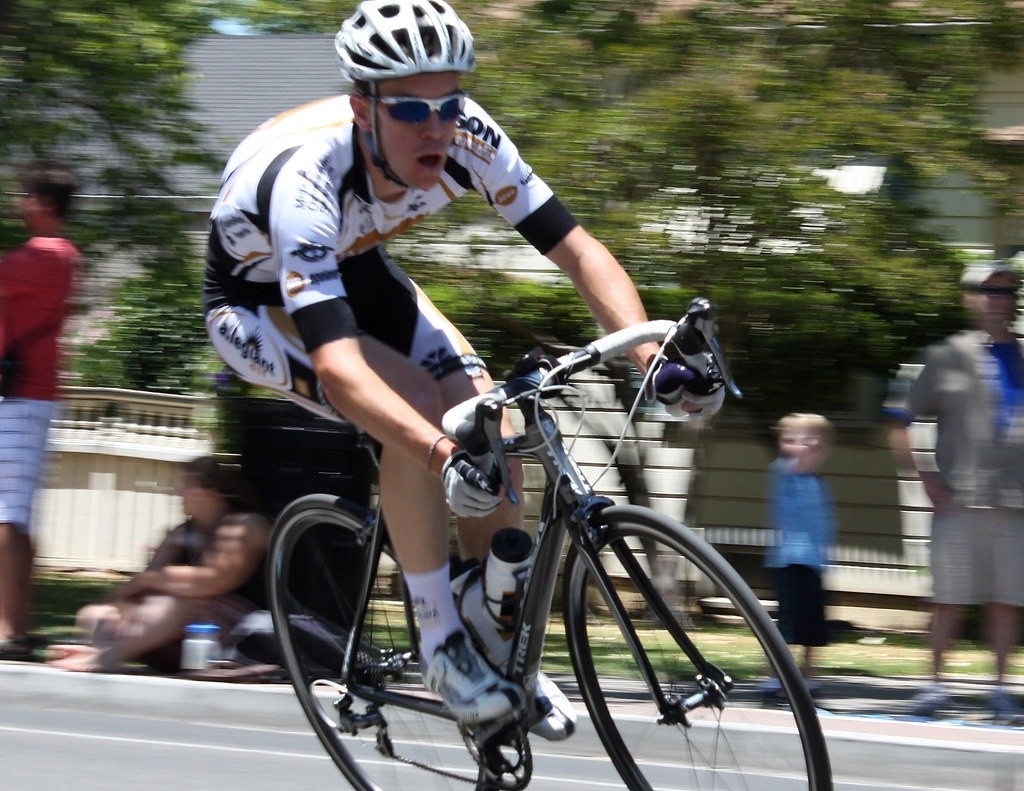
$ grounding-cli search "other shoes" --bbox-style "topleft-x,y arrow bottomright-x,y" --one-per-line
0,635 -> 33,661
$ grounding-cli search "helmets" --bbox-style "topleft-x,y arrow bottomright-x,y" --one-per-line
335,1 -> 479,82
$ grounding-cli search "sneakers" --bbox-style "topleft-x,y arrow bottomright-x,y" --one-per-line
421,634 -> 524,724
519,670 -> 577,741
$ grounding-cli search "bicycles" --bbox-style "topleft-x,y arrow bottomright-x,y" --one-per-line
260,294 -> 833,791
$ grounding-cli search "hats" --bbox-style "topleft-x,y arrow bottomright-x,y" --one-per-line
961,261 -> 1017,287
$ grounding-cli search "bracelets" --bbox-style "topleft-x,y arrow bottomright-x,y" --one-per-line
426,434 -> 447,472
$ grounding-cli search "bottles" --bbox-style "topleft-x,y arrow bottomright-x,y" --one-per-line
449,555 -> 515,676
482,527 -> 533,627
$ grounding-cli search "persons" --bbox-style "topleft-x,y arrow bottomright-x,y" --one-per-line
880,259 -> 1023,712
201,1 -> 725,740
0,169 -> 85,661
51,453 -> 273,672
761,415 -> 837,696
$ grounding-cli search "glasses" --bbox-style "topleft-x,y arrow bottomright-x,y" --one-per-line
963,284 -> 1018,298
355,90 -> 472,122
